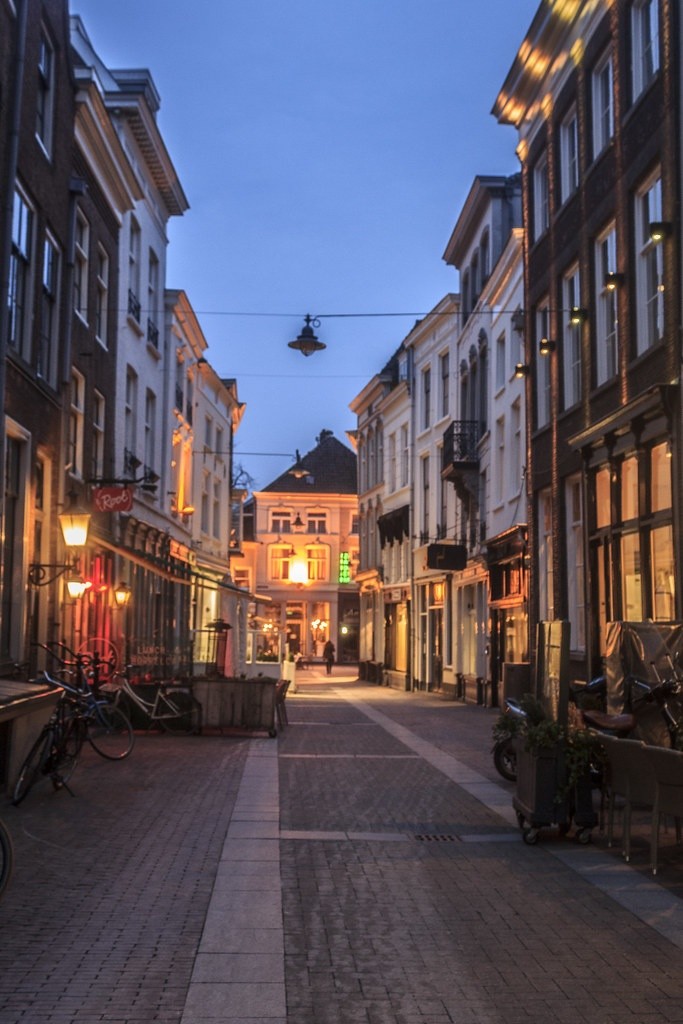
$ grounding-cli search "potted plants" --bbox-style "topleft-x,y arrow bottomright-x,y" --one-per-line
490,692 -> 609,843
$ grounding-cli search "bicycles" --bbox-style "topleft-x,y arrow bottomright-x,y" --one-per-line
9,641 -> 136,807
99,664 -> 201,737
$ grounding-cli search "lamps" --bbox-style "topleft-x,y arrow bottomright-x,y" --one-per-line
290,513 -> 306,530
61,576 -> 86,610
27,485 -> 92,586
289,462 -> 310,479
288,314 -> 326,356
108,581 -> 132,614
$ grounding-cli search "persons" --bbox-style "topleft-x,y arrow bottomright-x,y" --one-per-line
323,640 -> 336,675
256,645 -> 278,662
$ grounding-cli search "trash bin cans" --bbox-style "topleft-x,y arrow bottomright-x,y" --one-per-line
367,661 -> 382,682
358,660 -> 369,681
502,662 -> 533,697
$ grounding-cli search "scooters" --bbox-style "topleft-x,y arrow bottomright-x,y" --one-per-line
581,676 -> 683,752
489,699 -> 526,783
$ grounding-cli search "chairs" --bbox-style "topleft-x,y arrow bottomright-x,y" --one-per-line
276,679 -> 292,732
